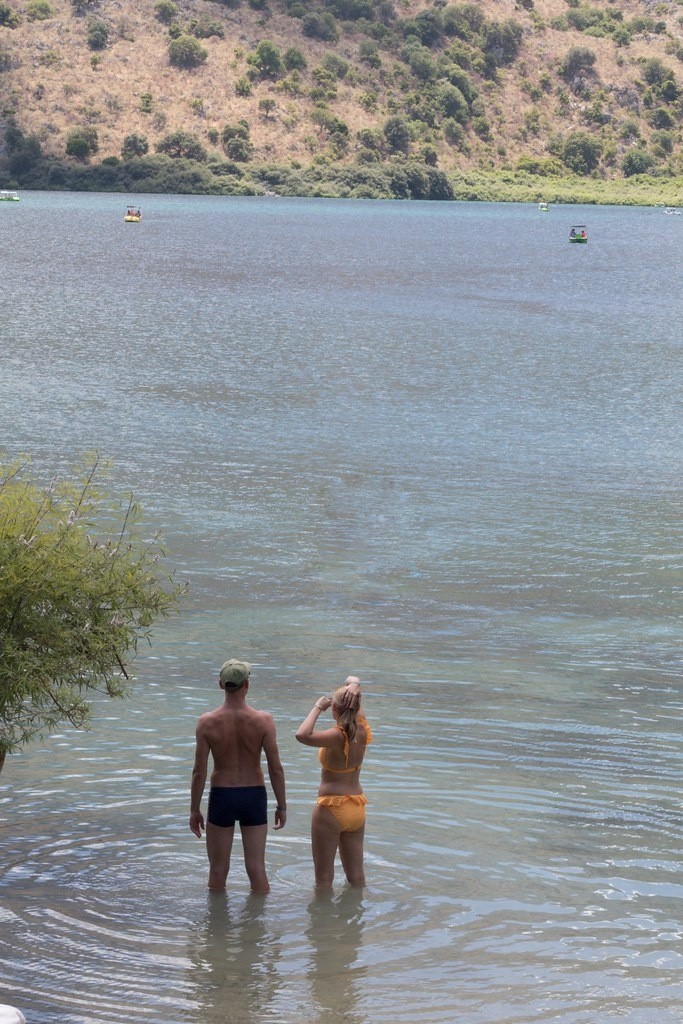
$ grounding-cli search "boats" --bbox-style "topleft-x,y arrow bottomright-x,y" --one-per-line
569,225 -> 587,243
538,203 -> 550,212
124,205 -> 142,223
0,191 -> 20,201
663,208 -> 681,215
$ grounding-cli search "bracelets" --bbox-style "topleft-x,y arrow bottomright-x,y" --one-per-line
315,704 -> 322,711
351,681 -> 360,686
276,806 -> 286,811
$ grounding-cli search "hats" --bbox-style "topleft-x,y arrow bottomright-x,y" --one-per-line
219,658 -> 251,689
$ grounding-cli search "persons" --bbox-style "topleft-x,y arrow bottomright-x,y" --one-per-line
295,676 -> 373,884
571,229 -> 576,238
127,211 -> 140,216
582,231 -> 586,237
189,658 -> 287,894
